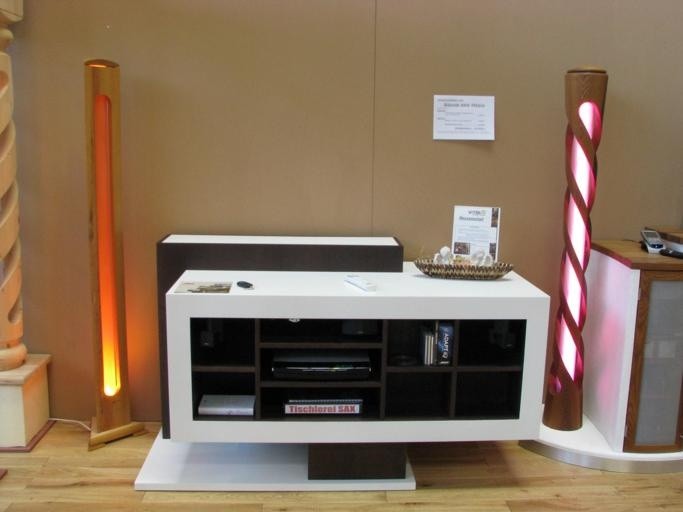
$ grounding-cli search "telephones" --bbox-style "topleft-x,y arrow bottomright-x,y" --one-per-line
641,229 -> 666,254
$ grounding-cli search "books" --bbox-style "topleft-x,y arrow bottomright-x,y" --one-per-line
452,204 -> 501,264
391,322 -> 454,365
284,398 -> 363,415
198,394 -> 256,416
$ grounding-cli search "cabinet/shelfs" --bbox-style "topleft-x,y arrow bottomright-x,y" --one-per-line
133,234 -> 550,491
517,240 -> 683,473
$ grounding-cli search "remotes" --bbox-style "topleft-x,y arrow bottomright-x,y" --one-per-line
660,249 -> 682,259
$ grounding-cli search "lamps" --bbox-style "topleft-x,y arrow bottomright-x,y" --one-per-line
541,67 -> 609,430
86,60 -> 143,445
